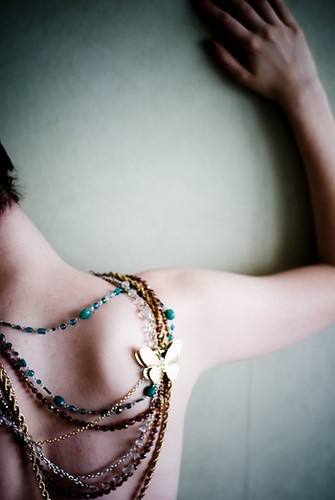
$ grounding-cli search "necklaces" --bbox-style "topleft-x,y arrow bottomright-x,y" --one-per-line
1,270 -> 176,500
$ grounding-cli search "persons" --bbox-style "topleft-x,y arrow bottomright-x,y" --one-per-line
2,0 -> 335,498
